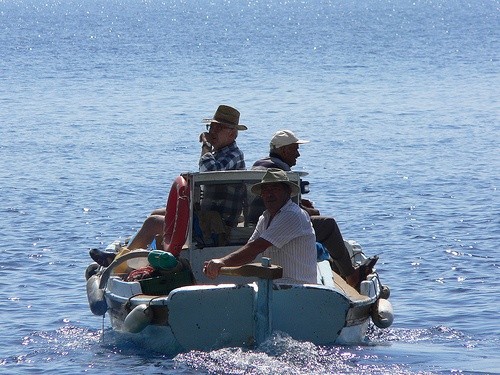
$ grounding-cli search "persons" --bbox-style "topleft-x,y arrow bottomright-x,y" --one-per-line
89,105 -> 247,266
244,131 -> 379,288
204,167 -> 320,285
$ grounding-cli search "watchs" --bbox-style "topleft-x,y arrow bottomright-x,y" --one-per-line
201,141 -> 212,149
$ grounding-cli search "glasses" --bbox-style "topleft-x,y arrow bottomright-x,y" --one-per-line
206,124 -> 227,132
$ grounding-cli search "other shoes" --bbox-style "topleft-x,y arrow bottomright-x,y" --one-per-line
362,256 -> 379,270
89,249 -> 116,266
345,265 -> 373,291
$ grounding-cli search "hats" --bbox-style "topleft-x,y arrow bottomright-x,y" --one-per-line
207,105 -> 247,130
251,168 -> 301,197
270,130 -> 309,150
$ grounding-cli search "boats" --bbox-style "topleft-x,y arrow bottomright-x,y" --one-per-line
86,168 -> 396,352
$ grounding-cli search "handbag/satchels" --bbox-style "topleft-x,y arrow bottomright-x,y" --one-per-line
190,210 -> 228,249
